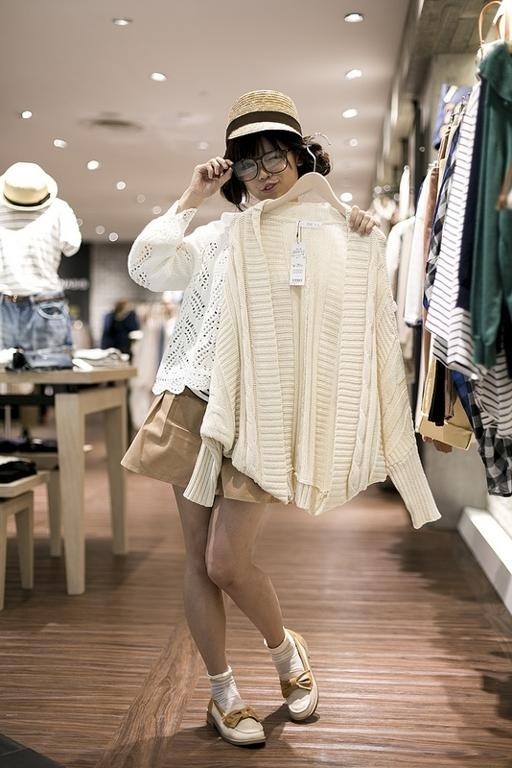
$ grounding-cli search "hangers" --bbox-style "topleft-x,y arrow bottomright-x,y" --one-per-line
262,133 -> 351,218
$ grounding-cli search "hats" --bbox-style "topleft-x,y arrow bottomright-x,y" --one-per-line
1,161 -> 58,211
225,90 -> 303,140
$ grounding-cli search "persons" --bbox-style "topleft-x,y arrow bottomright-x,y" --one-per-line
0,160 -> 86,372
122,90 -> 379,744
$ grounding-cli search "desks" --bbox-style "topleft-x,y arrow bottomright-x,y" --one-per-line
0,367 -> 138,596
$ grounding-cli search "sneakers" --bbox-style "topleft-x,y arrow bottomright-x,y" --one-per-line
207,695 -> 267,745
278,629 -> 319,720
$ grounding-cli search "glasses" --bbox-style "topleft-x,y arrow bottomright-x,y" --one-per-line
229,147 -> 295,182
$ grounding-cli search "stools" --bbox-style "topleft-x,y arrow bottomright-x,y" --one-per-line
3,444 -> 93,557
0,471 -> 52,610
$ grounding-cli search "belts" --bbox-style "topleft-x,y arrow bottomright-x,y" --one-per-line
0,294 -> 66,303
